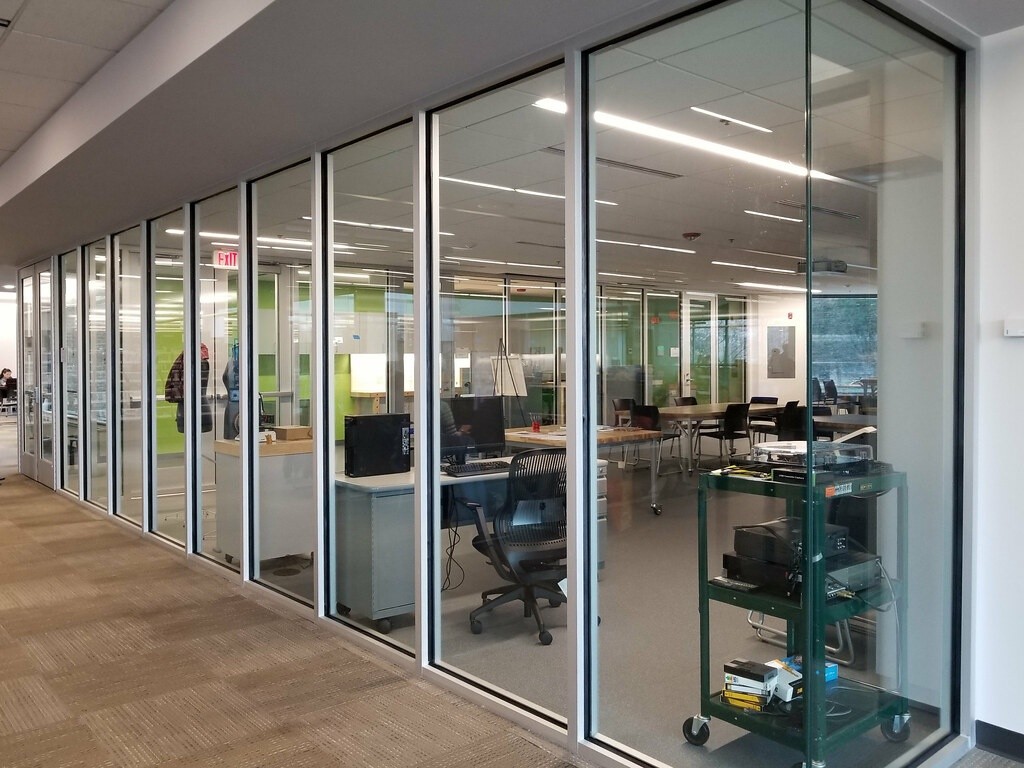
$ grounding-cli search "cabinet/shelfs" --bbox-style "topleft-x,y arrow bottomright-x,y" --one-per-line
682,463 -> 912,768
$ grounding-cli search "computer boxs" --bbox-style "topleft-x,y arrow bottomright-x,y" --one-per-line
344,413 -> 410,478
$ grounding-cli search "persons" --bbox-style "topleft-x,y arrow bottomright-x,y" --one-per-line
0,368 -> 12,386
440,400 -> 476,446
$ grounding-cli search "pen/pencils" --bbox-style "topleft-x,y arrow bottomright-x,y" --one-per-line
529,412 -> 541,421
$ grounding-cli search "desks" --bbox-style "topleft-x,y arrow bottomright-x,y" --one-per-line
335,454 -> 608,635
213,438 -> 315,571
614,402 -> 806,477
505,422 -> 663,516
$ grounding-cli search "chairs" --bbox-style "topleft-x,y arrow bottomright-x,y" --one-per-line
729,397 -> 778,456
694,403 -> 753,473
776,377 -> 877,442
747,400 -> 800,455
623,405 -> 684,476
453,446 -> 602,646
607,399 -> 661,466
669,397 -> 724,461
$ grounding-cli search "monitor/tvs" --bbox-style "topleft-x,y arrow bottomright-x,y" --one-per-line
440,395 -> 506,456
5,378 -> 17,390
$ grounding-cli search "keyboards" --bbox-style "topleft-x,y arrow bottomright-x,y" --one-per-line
441,461 -> 527,478
